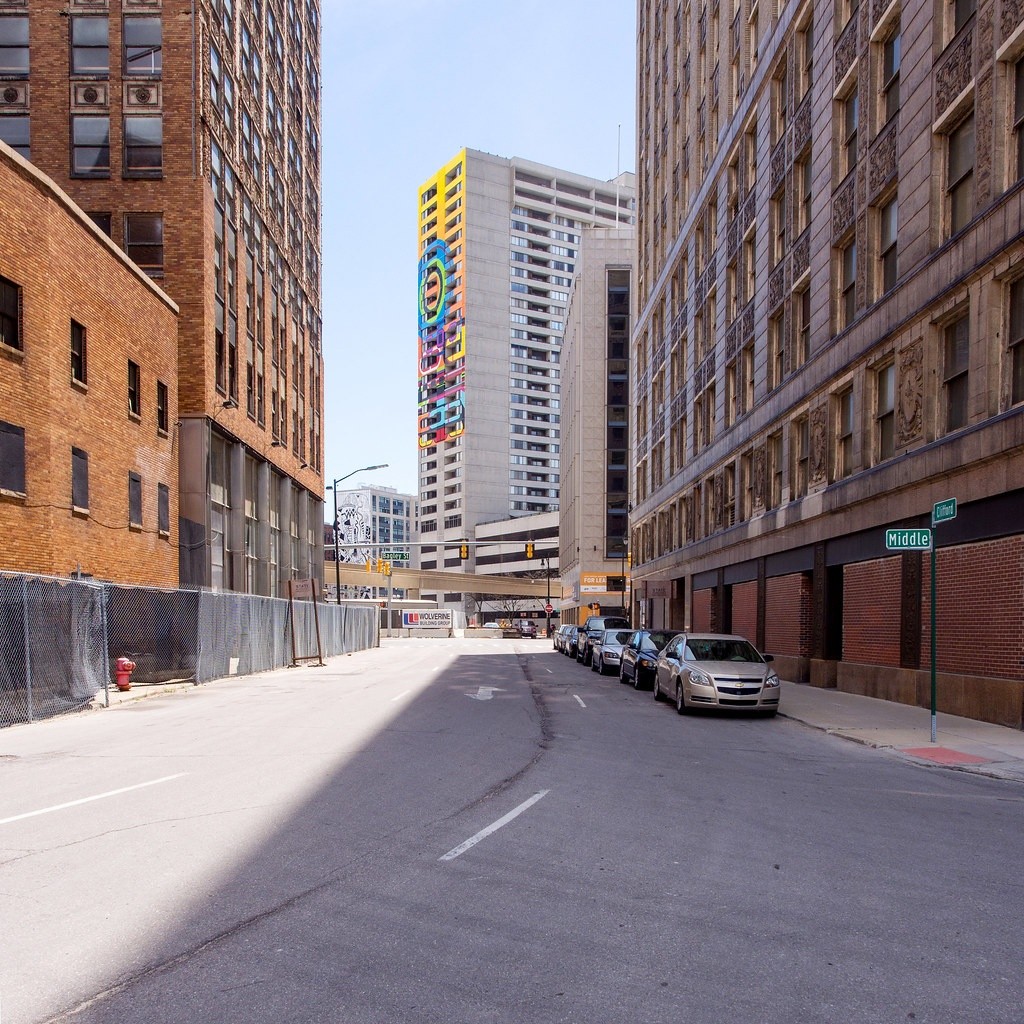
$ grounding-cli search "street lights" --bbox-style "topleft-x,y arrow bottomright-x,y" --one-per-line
621,531 -> 631,618
541,554 -> 552,637
333,463 -> 390,608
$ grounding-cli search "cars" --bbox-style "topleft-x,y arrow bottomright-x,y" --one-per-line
553,623 -> 584,658
654,632 -> 784,717
620,629 -> 688,690
592,628 -> 637,675
517,620 -> 539,639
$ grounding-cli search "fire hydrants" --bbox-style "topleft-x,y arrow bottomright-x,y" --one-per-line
115,657 -> 138,692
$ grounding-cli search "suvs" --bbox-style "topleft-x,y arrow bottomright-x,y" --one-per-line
576,616 -> 632,666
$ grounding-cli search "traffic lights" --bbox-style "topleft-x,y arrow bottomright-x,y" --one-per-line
591,603 -> 600,610
378,558 -> 383,573
385,562 -> 392,576
380,602 -> 387,607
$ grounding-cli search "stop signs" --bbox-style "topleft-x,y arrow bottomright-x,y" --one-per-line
545,604 -> 554,614
